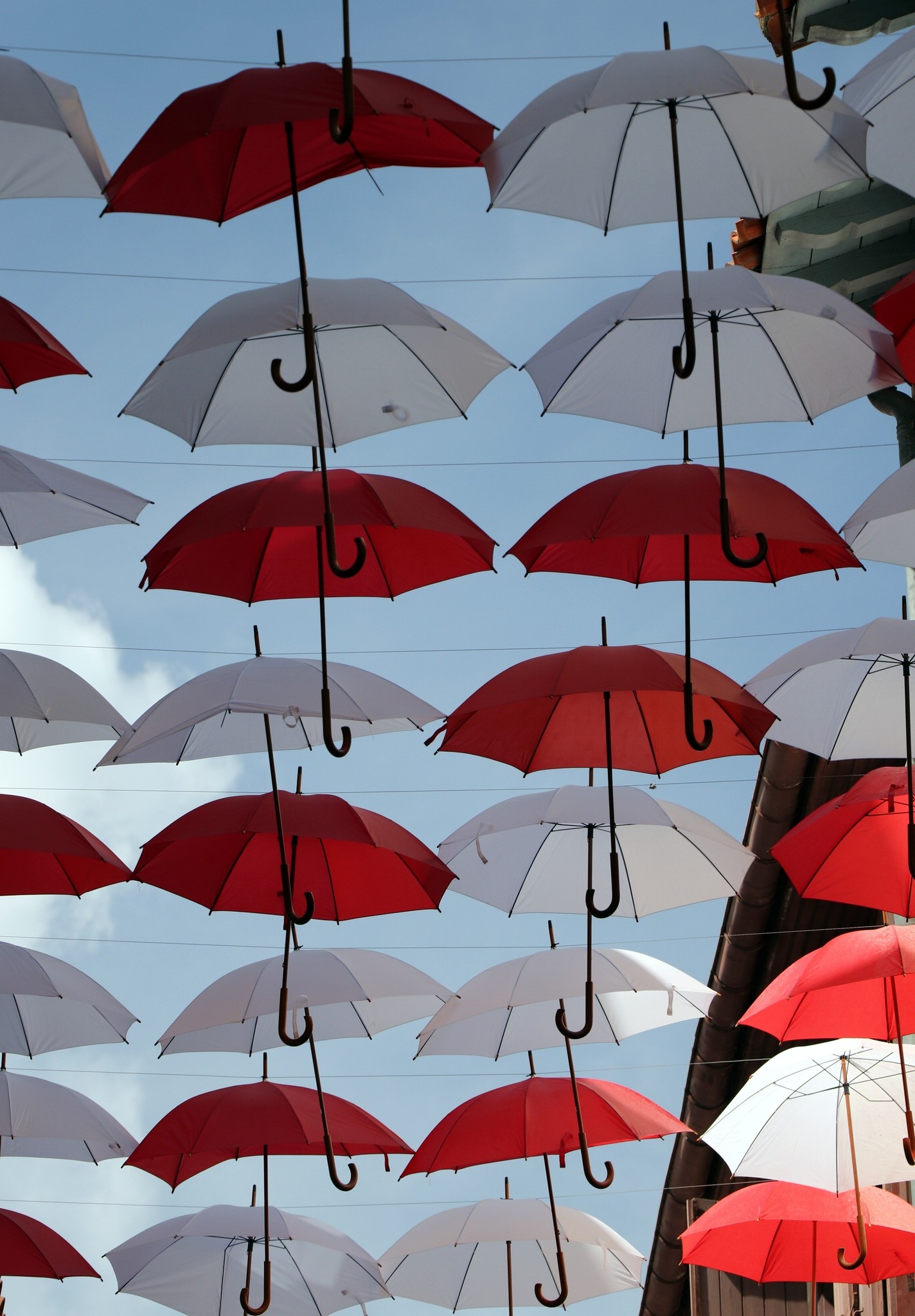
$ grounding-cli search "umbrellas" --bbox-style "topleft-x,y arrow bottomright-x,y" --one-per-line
0,0 -> 915,1316
